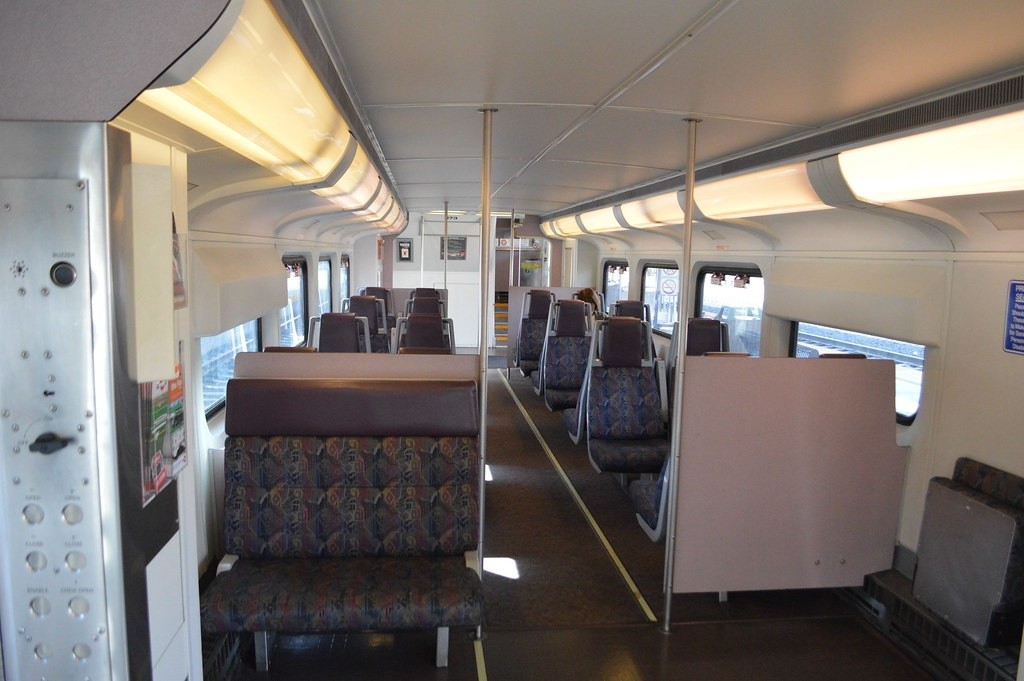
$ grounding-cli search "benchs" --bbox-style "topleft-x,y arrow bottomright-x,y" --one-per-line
199,377 -> 484,673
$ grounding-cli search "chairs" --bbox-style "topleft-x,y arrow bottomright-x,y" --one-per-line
609,301 -> 657,361
307,313 -> 372,355
564,335 -> 655,445
340,295 -> 390,354
586,317 -> 667,495
516,290 -> 540,378
401,297 -> 448,333
409,288 -> 441,303
389,312 -> 457,356
543,301 -> 583,413
666,317 -> 728,397
359,287 -> 395,317
